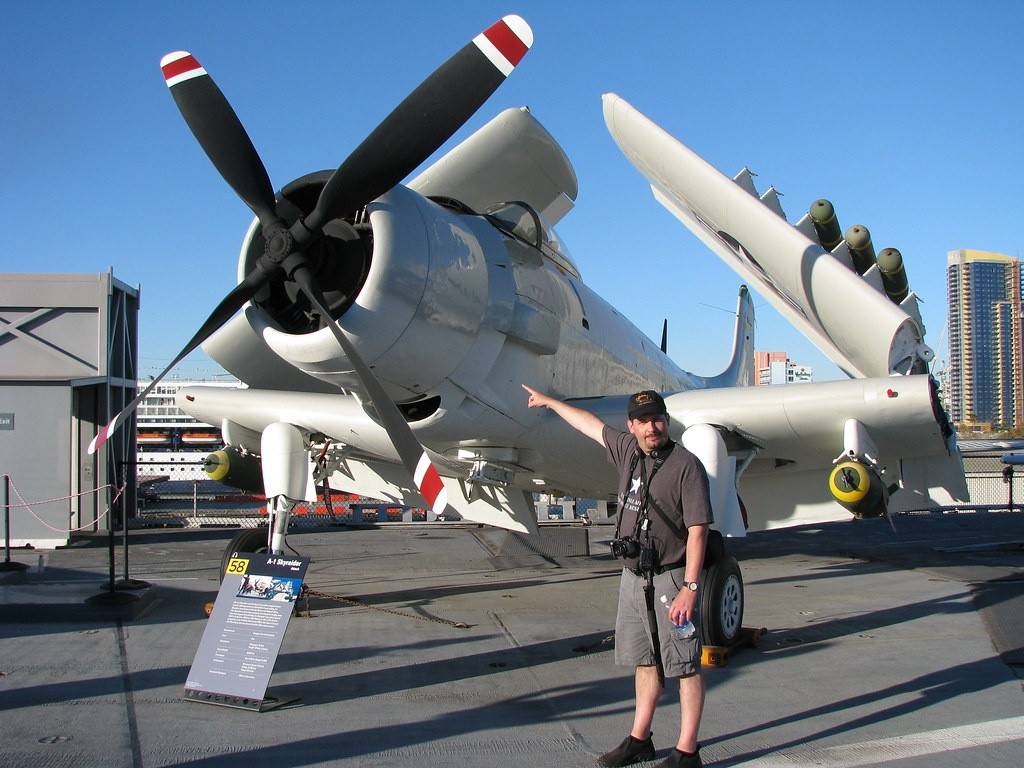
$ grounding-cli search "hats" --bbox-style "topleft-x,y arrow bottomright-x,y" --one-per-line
627,390 -> 666,417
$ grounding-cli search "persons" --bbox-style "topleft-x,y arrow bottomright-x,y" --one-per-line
522,383 -> 715,768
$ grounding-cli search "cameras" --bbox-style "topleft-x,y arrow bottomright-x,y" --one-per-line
609,536 -> 640,559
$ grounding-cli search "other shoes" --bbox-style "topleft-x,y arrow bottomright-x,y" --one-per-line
655,742 -> 703,768
597,731 -> 656,767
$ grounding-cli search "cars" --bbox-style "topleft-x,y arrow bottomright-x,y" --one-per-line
579,514 -> 592,526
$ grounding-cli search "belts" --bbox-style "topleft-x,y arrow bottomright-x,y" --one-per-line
624,561 -> 687,579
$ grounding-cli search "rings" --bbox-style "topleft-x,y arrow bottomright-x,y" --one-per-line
679,613 -> 685,616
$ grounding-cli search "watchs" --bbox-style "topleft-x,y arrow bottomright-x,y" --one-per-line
682,581 -> 698,592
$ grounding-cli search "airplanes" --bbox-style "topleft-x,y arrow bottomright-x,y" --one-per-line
86,15 -> 971,588
